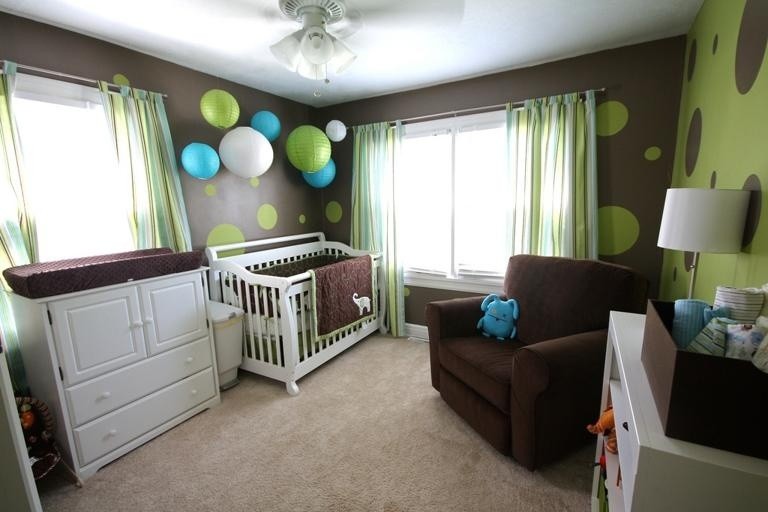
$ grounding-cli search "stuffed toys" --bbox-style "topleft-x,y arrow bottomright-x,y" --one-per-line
477,293 -> 520,343
587,405 -> 618,456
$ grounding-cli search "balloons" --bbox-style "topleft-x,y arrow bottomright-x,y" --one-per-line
285,125 -> 332,174
199,88 -> 240,129
250,109 -> 282,144
181,141 -> 220,181
219,127 -> 274,179
301,158 -> 336,189
326,119 -> 347,143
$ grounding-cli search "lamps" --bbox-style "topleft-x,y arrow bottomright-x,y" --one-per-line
267,0 -> 354,81
655,185 -> 752,299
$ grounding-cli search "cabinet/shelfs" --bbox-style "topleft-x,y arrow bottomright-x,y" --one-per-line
5,267 -> 222,483
587,310 -> 767,512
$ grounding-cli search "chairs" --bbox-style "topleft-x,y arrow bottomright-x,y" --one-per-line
421,253 -> 648,473
13,396 -> 84,489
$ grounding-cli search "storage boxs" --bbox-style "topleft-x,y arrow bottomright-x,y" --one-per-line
639,299 -> 767,461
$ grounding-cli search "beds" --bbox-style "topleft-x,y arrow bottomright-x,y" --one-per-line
203,231 -> 388,396
